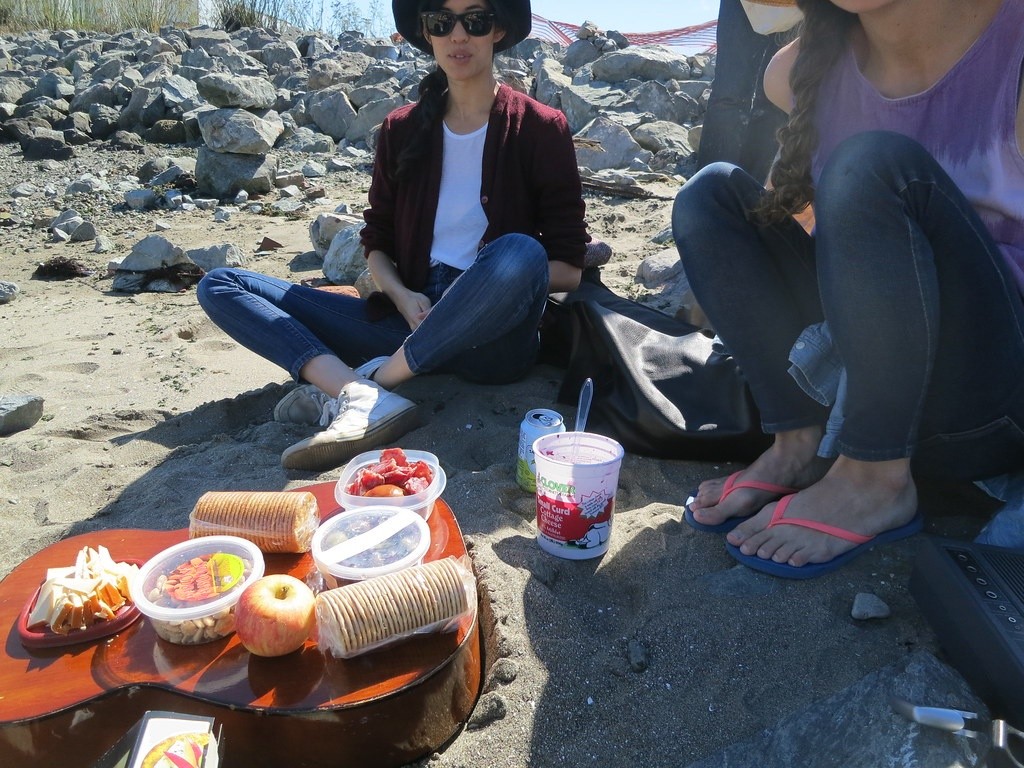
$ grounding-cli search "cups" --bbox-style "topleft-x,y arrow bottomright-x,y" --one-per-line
532,432 -> 624,561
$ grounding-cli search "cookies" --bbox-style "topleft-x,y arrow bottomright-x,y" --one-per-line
316,558 -> 471,658
190,491 -> 318,553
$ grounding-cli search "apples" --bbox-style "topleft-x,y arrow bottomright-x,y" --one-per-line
236,574 -> 315,656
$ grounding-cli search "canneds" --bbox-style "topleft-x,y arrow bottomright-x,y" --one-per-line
516,408 -> 565,494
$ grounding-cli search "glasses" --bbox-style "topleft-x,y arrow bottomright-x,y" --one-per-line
421,10 -> 497,37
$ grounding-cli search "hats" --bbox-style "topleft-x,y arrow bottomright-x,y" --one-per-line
391,0 -> 531,57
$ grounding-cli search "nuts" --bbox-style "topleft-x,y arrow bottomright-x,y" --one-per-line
148,574 -> 249,645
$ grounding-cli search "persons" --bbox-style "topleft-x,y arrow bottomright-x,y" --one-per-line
198,0 -> 587,469
672,0 -> 1024,577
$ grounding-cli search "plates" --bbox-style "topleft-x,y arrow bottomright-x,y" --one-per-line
19,558 -> 151,649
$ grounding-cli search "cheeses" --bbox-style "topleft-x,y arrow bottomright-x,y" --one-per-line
27,545 -> 139,635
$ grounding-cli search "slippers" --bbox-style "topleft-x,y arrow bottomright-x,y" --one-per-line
725,492 -> 924,579
685,470 -> 799,533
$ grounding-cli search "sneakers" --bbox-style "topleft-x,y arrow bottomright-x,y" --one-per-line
281,393 -> 418,471
274,357 -> 401,426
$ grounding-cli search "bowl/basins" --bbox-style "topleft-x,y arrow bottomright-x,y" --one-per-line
311,505 -> 431,590
131,534 -> 266,647
334,448 -> 447,524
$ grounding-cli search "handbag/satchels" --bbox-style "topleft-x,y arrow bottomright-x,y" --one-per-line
573,283 -> 752,465
